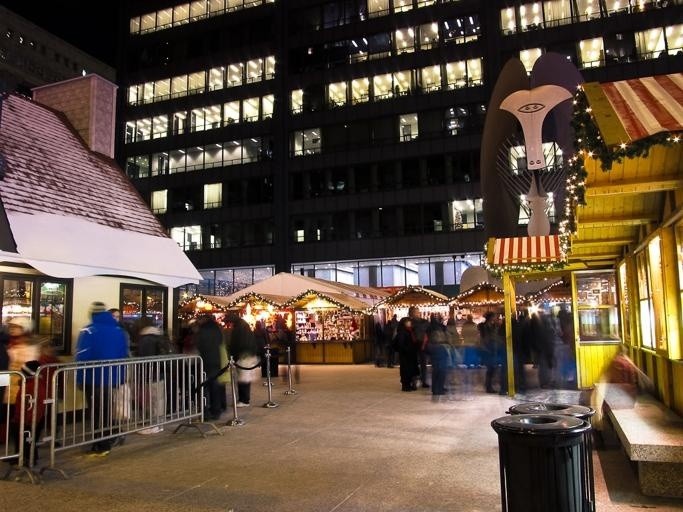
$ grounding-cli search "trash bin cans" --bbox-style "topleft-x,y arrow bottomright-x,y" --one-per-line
491,402 -> 596,512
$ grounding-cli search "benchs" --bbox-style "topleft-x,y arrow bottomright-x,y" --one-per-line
594,381 -> 683,499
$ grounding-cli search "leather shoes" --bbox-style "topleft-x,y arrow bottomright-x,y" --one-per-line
91,442 -> 113,452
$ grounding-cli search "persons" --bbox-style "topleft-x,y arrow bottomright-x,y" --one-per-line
0,330 -> 11,411
36,343 -> 65,446
107,307 -> 132,446
2,316 -> 36,443
0,360 -> 46,464
372,303 -> 577,401
182,303 -> 289,422
132,316 -> 174,434
72,300 -> 128,456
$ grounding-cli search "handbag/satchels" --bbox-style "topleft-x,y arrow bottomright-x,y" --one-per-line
113,383 -> 131,421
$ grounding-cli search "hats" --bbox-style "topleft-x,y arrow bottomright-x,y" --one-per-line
21,361 -> 42,376
88,301 -> 106,316
8,316 -> 33,332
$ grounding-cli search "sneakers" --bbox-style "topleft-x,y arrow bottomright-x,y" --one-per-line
237,402 -> 250,408
136,427 -> 159,434
